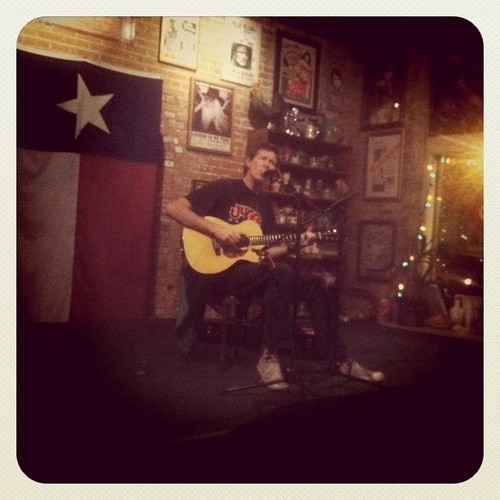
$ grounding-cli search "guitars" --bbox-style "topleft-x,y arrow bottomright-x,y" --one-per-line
182,215 -> 340,276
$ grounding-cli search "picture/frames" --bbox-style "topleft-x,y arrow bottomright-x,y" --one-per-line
274,27 -> 323,112
360,52 -> 409,131
188,77 -> 234,154
364,132 -> 404,200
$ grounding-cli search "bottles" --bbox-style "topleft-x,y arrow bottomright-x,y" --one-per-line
270,108 -> 340,199
277,205 -> 338,232
449,299 -> 464,325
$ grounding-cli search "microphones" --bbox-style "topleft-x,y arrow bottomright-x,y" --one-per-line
263,168 -> 279,176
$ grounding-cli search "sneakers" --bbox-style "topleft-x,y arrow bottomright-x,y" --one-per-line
335,359 -> 384,382
257,358 -> 288,390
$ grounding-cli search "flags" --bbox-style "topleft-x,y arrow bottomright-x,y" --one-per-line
17,45 -> 162,322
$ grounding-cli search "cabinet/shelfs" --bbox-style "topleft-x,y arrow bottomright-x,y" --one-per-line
246,129 -> 353,351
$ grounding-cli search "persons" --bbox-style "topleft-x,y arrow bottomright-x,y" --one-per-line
231,44 -> 252,70
164,140 -> 385,393
194,90 -> 230,134
286,52 -> 313,100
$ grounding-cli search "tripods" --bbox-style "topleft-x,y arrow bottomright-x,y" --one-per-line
225,173 -> 389,407
